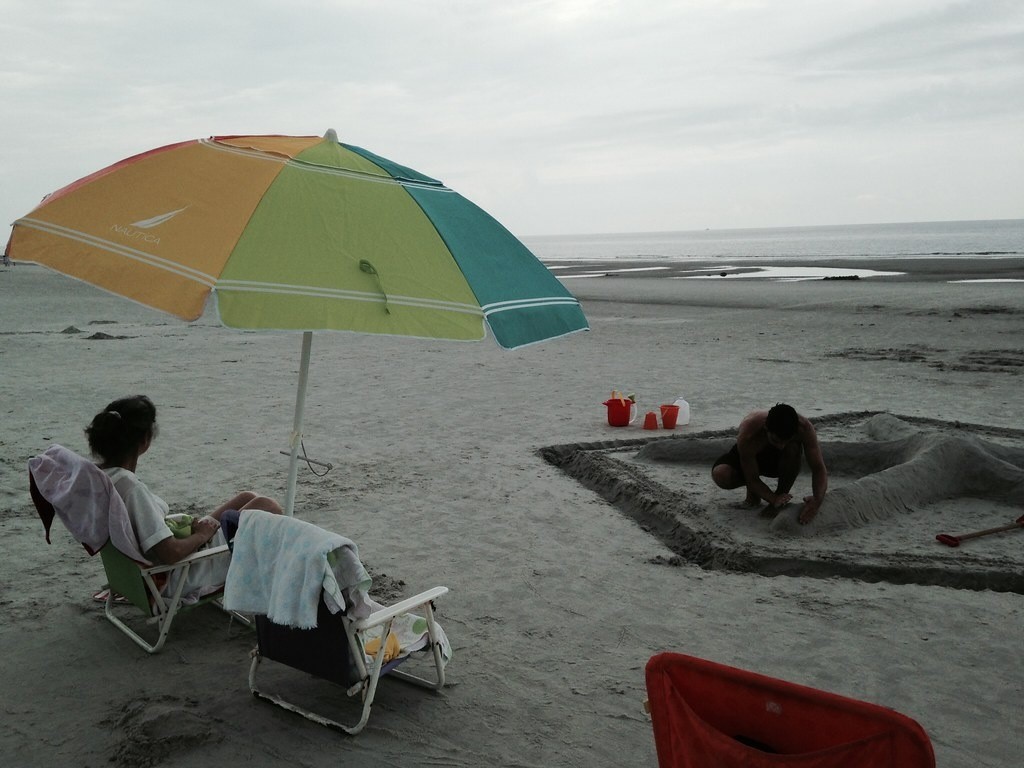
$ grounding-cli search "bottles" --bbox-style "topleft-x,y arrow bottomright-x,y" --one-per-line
673,397 -> 690,426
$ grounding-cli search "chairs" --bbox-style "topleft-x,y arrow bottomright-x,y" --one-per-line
218,509 -> 450,733
643,652 -> 938,768
28,446 -> 241,654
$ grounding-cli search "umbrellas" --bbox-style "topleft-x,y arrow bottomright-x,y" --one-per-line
2,131 -> 592,515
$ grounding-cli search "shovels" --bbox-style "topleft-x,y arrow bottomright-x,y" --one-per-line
935,514 -> 1024,548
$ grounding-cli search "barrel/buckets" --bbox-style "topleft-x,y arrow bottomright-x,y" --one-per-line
659,404 -> 680,429
603,390 -> 637,427
643,412 -> 658,429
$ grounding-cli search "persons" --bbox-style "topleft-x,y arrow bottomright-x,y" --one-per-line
82,393 -> 283,603
710,401 -> 828,526
